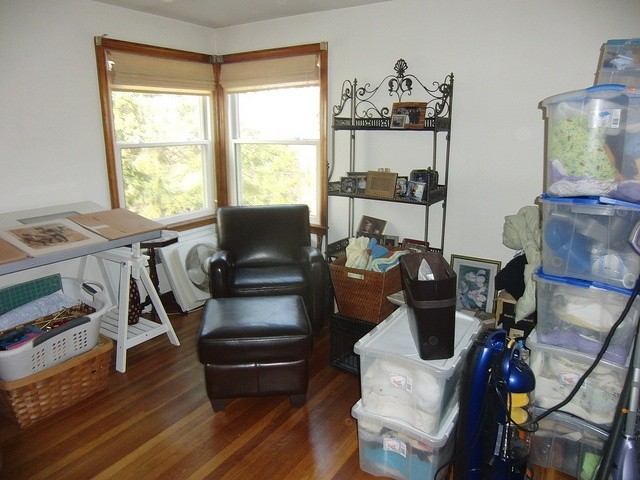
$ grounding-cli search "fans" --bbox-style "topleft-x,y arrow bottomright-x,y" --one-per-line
158,228 -> 219,312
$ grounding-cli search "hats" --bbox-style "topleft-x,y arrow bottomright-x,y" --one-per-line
476,270 -> 486,277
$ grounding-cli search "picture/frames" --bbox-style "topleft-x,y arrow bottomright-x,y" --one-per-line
449,254 -> 500,315
389,113 -> 406,129
391,102 -> 427,129
356,215 -> 429,252
338,169 -> 428,202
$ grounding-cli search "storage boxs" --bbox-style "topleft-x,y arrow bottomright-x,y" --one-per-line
494,37 -> 640,480
329,313 -> 378,377
354,304 -> 485,437
353,398 -> 461,480
0,277 -> 111,382
402,251 -> 458,361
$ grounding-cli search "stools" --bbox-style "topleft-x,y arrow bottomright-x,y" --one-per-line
126,229 -> 180,321
197,295 -> 313,400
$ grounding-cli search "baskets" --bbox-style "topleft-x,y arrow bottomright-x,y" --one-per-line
0,333 -> 114,430
329,246 -> 416,325
0,276 -> 110,383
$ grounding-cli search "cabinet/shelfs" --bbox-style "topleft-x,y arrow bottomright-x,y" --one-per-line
325,58 -> 455,315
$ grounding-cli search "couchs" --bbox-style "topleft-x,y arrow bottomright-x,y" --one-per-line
207,202 -> 327,334
